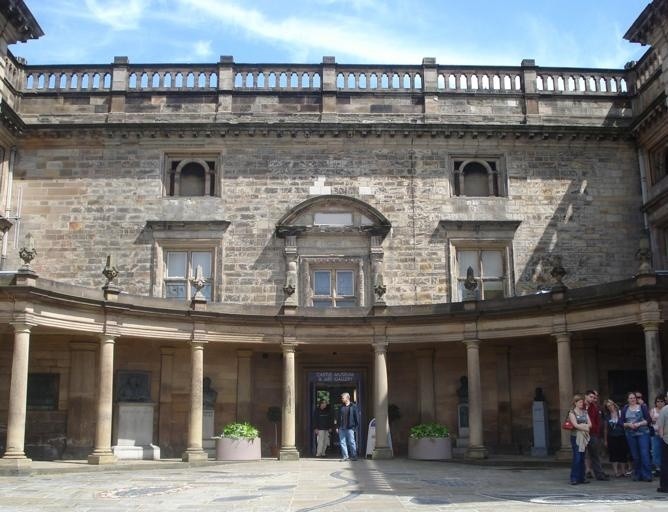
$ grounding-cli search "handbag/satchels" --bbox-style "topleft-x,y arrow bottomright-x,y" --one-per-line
562,420 -> 576,430
650,426 -> 656,437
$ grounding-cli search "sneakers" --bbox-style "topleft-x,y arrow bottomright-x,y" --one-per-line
570,467 -> 668,493
316,453 -> 358,463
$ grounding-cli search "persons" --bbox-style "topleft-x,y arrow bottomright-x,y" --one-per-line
313,398 -> 334,457
117,377 -> 146,401
532,388 -> 544,401
334,392 -> 360,463
202,377 -> 218,412
562,388 -> 668,494
454,373 -> 467,400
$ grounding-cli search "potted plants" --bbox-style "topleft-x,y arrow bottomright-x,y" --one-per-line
216,421 -> 261,460
408,422 -> 451,460
